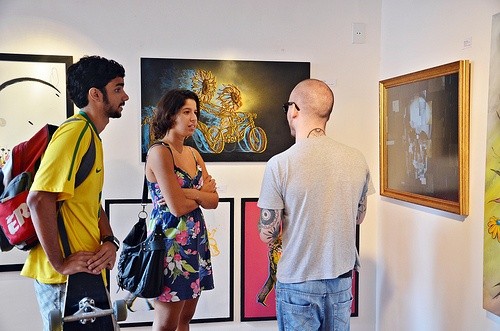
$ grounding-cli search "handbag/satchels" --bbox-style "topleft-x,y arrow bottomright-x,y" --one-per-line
116,218 -> 166,298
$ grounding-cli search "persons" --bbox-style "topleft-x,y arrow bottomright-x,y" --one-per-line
145,89 -> 219,331
409,97 -> 431,155
20,54 -> 129,331
256,79 -> 377,331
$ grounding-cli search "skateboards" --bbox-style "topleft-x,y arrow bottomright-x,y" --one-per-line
48,272 -> 114,331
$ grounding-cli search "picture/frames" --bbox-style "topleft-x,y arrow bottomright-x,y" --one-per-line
241,198 -> 360,321
105,198 -> 234,327
0,53 -> 74,272
379,60 -> 469,216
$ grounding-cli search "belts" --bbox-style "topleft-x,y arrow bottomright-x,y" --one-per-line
339,271 -> 353,278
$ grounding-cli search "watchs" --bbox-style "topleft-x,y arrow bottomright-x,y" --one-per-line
103,236 -> 120,251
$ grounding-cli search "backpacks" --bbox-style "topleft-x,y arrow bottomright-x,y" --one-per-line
0,119 -> 96,252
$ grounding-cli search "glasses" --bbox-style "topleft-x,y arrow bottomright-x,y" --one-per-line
282,102 -> 300,113
